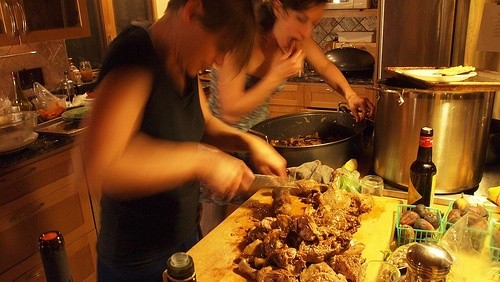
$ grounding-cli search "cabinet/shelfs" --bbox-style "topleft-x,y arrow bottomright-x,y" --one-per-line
0,97 -> 92,278
0,0 -> 93,49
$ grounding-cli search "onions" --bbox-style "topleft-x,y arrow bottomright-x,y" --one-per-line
399,193 -> 489,241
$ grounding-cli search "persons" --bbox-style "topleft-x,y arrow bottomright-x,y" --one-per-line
197,1 -> 377,243
78,1 -> 288,282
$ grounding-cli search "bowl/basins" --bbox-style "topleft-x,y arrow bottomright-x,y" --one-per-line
1,112 -> 35,152
31,95 -> 68,122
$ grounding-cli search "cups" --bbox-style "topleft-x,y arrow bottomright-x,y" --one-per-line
79,60 -> 93,82
360,175 -> 385,221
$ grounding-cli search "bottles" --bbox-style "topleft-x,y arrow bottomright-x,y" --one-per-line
407,126 -> 437,209
61,57 -> 81,103
40,228 -> 74,282
166,251 -> 196,282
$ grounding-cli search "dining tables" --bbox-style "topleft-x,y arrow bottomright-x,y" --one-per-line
159,182 -> 500,282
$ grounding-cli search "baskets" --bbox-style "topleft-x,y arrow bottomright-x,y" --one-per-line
488,220 -> 500,261
394,201 -> 445,247
442,201 -> 494,253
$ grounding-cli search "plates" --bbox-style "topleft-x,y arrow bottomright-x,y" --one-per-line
62,107 -> 85,121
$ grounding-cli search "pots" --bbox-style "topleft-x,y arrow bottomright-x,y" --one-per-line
364,77 -> 495,195
322,43 -> 374,79
240,101 -> 374,174
